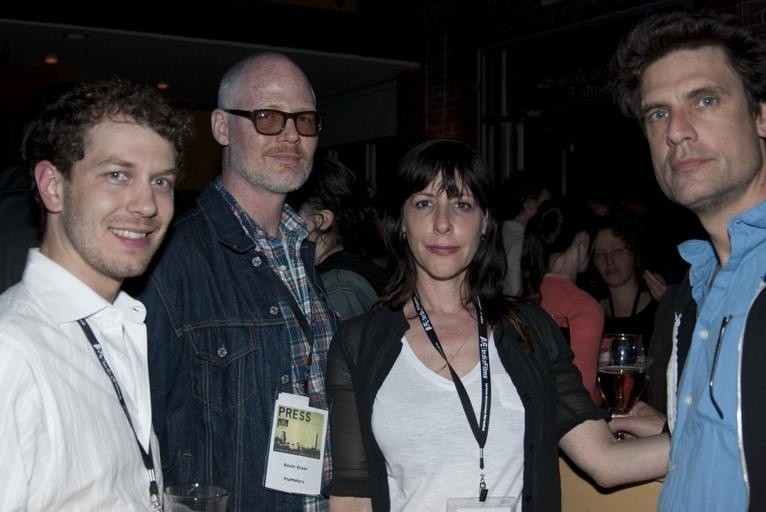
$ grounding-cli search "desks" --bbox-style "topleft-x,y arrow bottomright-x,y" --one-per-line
560,454 -> 665,510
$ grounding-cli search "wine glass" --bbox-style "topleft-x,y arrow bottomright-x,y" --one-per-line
595,332 -> 648,443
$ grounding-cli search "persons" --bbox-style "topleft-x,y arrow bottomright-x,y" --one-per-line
283,157 -> 678,424
0,75 -> 197,510
605,14 -> 766,512
134,50 -> 342,512
326,139 -> 672,512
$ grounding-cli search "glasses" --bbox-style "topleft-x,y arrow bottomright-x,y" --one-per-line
225,108 -> 321,137
